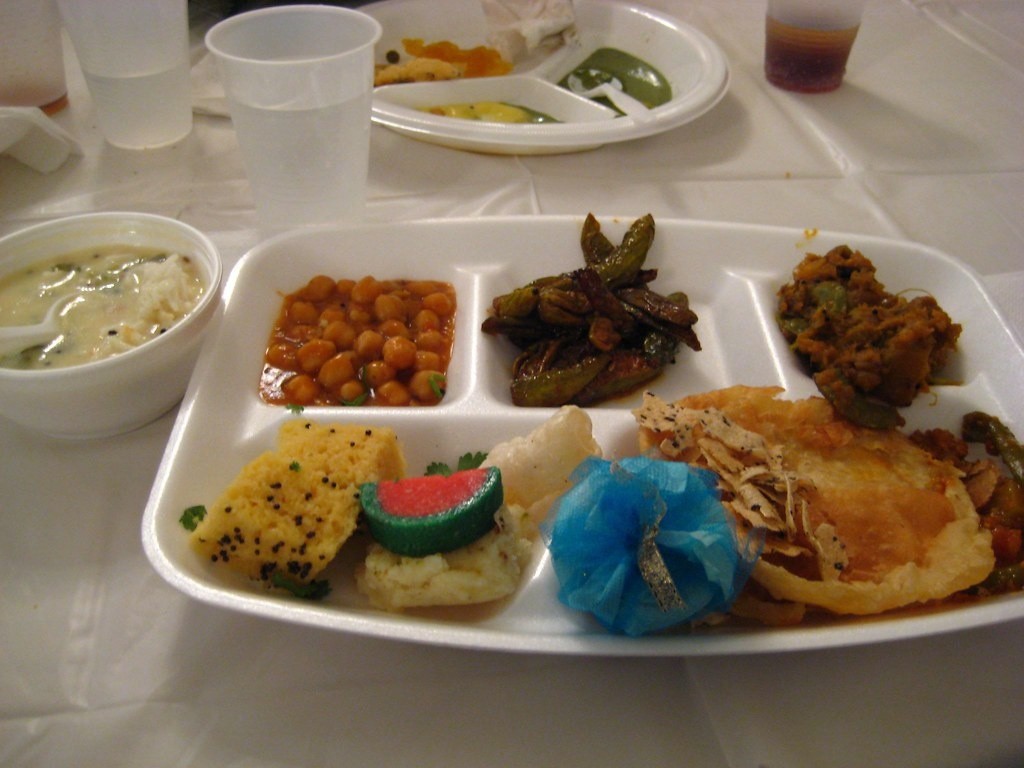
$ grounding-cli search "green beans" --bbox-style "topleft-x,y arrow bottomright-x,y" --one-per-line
480,212 -> 702,409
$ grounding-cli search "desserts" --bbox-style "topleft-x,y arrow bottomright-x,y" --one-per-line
359,464 -> 504,557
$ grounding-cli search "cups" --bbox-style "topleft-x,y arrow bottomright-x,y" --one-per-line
763,0 -> 866,95
56,1 -> 194,151
204,5 -> 383,230
1,2 -> 68,118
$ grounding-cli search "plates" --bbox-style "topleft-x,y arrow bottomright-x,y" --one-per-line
136,213 -> 1024,658
354,0 -> 734,155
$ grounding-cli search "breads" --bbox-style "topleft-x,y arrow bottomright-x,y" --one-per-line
185,418 -> 408,593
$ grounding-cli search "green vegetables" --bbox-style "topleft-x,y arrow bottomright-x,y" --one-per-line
178,370 -> 489,601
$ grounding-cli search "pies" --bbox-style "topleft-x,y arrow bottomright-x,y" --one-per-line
628,383 -> 996,618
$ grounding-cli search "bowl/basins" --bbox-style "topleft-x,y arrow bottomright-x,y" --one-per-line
0,210 -> 224,443
